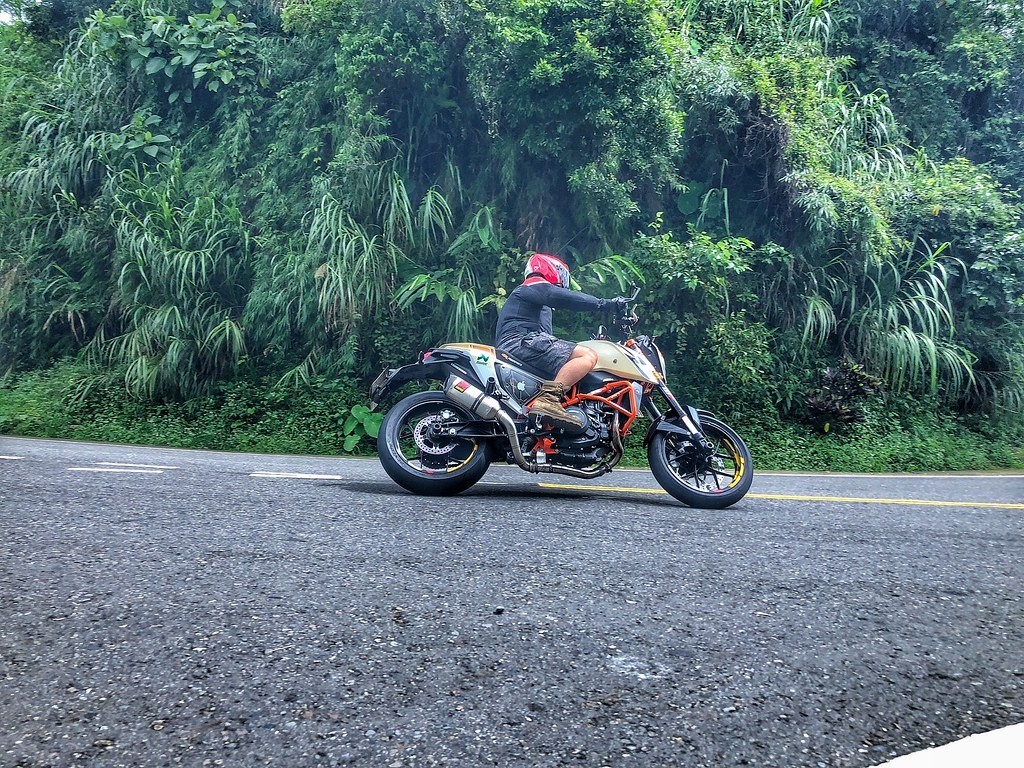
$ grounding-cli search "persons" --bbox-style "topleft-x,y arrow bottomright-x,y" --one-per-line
495,254 -> 629,431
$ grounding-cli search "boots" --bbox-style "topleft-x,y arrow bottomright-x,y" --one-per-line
529,381 -> 582,429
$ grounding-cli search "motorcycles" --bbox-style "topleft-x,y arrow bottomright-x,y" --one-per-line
368,280 -> 755,509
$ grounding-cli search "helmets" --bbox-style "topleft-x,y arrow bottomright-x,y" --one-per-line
524,253 -> 572,287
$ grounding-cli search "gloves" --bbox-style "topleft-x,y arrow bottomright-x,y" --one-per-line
610,296 -> 629,320
